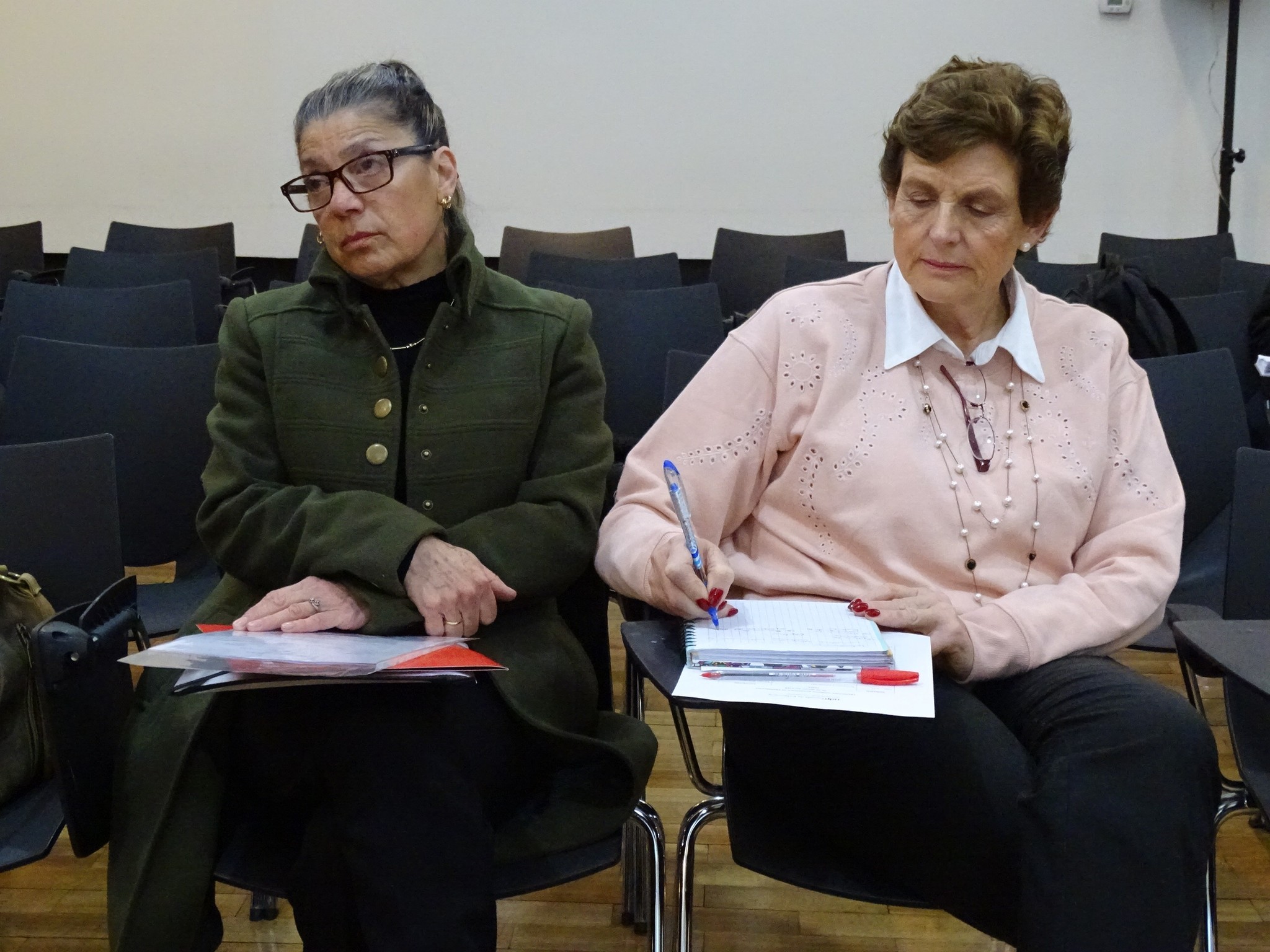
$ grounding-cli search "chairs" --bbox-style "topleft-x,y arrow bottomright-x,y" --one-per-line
1,221 -> 1270,952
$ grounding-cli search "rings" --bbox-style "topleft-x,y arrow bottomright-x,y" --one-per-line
306,595 -> 324,613
445,619 -> 464,627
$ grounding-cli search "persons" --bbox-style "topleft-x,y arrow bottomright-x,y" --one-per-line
104,57 -> 661,952
593,49 -> 1222,952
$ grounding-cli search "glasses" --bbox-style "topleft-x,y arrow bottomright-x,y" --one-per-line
939,362 -> 995,473
281,143 -> 459,213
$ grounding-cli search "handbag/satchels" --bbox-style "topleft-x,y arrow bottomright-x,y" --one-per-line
0,563 -> 57,799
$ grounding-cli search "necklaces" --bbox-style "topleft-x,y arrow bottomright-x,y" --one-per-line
388,295 -> 454,351
913,355 -> 1043,606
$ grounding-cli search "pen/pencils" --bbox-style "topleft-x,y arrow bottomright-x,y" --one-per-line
663,459 -> 720,630
700,670 -> 920,685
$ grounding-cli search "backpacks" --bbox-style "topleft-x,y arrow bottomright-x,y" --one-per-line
1061,252 -> 1199,359
